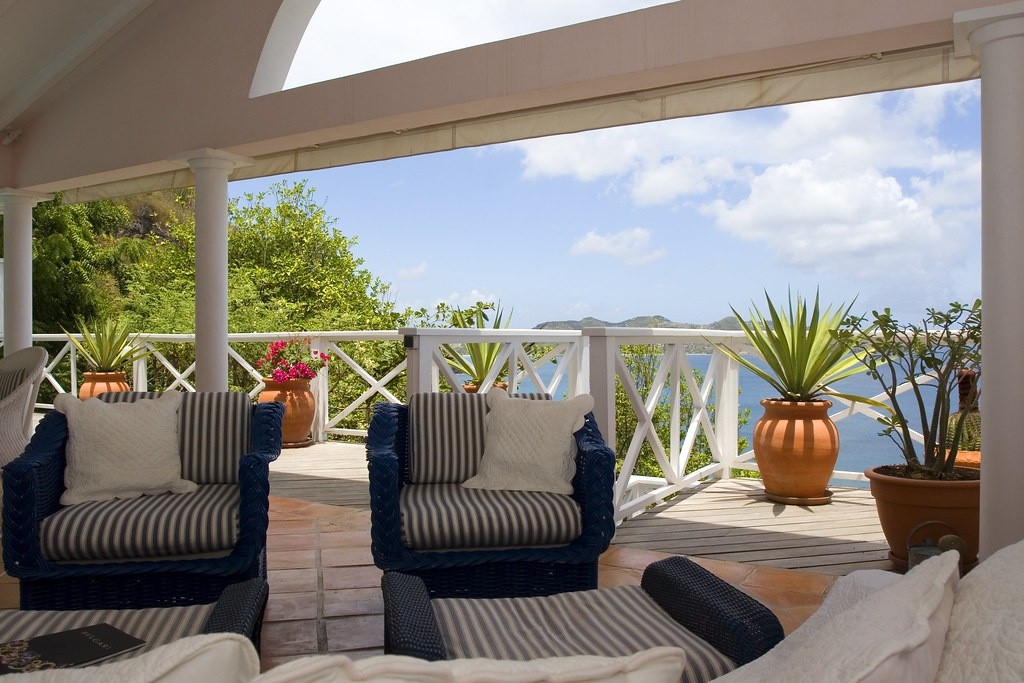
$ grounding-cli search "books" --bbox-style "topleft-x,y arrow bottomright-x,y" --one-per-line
0,623 -> 148,675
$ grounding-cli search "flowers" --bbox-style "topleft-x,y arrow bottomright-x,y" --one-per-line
256,333 -> 331,384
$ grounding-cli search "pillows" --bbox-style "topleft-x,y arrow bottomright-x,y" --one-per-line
461,386 -> 594,493
54,389 -> 197,505
716,539 -> 1023,682
0,633 -> 688,683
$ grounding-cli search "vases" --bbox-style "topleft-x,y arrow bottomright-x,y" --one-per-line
258,377 -> 315,442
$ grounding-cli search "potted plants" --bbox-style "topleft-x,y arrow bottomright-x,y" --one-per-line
830,300 -> 983,559
57,314 -> 162,401
431,299 -> 547,393
700,283 -> 899,498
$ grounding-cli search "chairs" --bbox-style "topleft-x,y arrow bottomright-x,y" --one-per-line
0,575 -> 267,683
0,386 -> 283,608
0,346 -> 49,537
365,391 -> 616,600
379,559 -> 785,683
